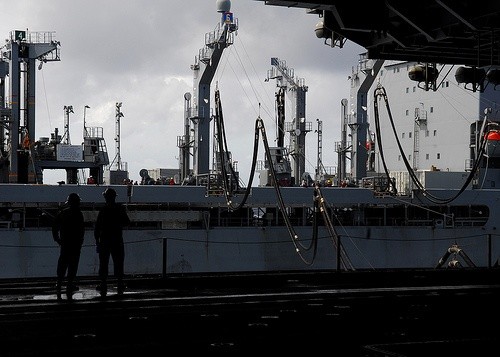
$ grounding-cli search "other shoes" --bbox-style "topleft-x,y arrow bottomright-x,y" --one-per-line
67,286 -> 79,290
55,283 -> 61,289
96,284 -> 107,289
118,282 -> 123,289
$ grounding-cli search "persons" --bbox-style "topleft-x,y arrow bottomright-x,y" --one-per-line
121,176 -> 175,185
299,174 -> 354,188
52,192 -> 85,299
93,187 -> 131,294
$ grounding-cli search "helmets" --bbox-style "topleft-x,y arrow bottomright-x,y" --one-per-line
69,193 -> 80,203
103,188 -> 117,198
